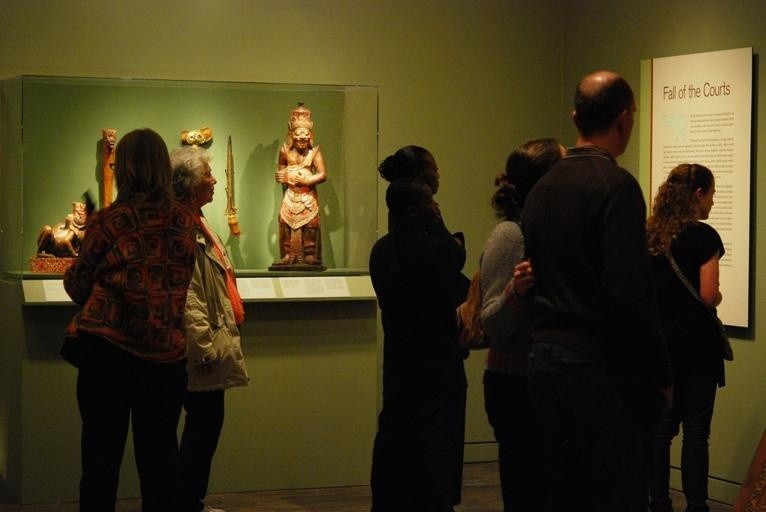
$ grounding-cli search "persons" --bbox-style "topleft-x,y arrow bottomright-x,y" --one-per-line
519,68 -> 676,512
266,102 -> 330,273
62,127 -> 200,511
476,136 -> 571,510
638,163 -> 736,512
163,146 -> 251,511
375,144 -> 489,507
365,176 -> 496,511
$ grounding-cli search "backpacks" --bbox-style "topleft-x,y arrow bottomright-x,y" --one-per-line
454,250 -> 490,351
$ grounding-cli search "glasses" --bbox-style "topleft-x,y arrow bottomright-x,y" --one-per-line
110,163 -> 118,170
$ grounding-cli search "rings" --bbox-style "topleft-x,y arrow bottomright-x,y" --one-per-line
520,270 -> 527,280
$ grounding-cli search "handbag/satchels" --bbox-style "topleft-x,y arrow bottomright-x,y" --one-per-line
715,317 -> 733,361
